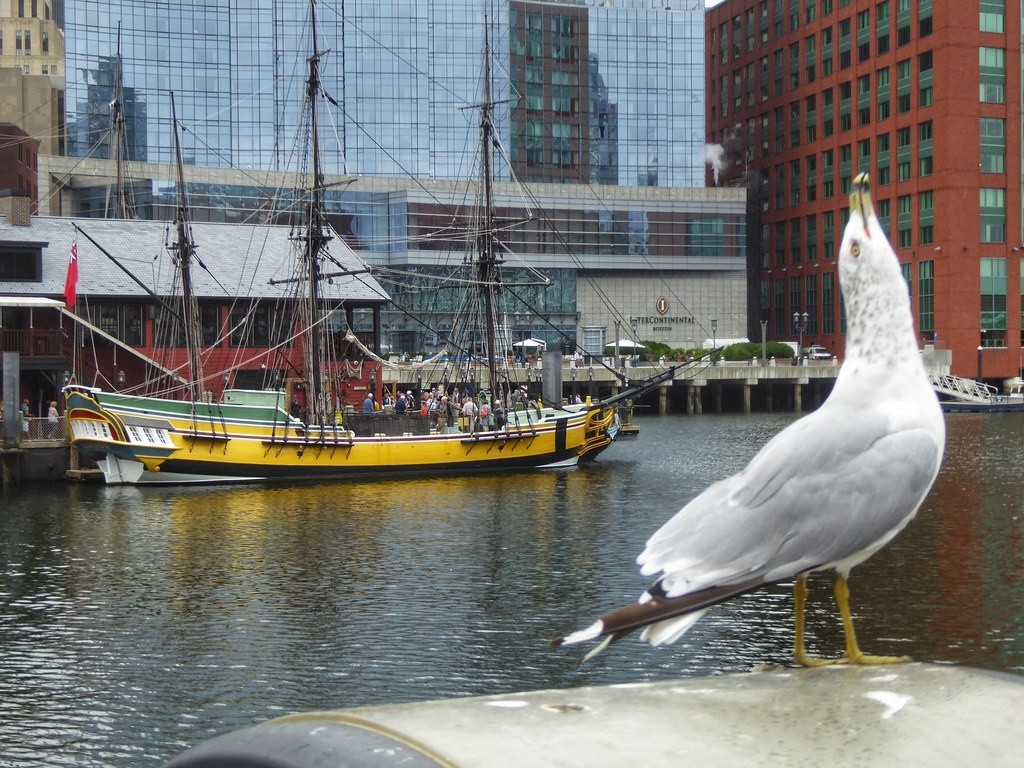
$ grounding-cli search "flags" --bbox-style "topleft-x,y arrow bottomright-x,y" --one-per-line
63,239 -> 78,307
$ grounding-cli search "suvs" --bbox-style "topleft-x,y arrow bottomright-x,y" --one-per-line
807,346 -> 833,361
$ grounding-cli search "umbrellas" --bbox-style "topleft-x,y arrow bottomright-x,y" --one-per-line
605,339 -> 645,347
513,338 -> 545,346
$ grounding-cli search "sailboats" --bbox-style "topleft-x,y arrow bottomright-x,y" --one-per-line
62,1 -> 724,489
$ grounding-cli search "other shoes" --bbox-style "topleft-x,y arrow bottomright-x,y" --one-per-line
50,438 -> 56,441
47,436 -> 51,439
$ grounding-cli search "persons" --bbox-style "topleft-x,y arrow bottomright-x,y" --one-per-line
404,352 -> 409,362
422,384 -> 503,432
573,350 -> 583,368
363,393 -> 376,415
290,394 -> 302,418
512,382 -> 528,408
791,355 -> 798,366
20,399 -> 33,440
383,385 -> 414,415
47,401 -> 59,439
0,400 -> 4,420
571,393 -> 582,404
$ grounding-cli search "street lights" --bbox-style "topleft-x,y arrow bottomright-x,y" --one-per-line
513,310 -> 532,369
711,320 -> 717,366
631,318 -> 638,366
793,312 -> 809,368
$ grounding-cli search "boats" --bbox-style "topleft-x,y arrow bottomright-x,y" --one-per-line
939,392 -> 1023,413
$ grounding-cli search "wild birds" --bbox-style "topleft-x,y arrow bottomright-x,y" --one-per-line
547,171 -> 948,668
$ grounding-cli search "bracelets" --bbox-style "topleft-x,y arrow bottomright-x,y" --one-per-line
30,414 -> 31,416
